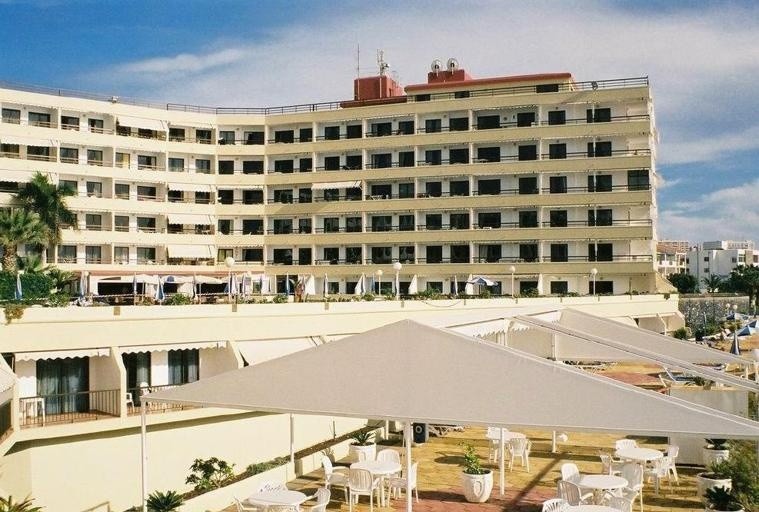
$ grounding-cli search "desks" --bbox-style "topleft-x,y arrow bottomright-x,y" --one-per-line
19,398 -> 45,427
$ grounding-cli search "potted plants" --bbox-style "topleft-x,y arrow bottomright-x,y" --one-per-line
347,427 -> 376,461
458,438 -> 494,503
696,438 -> 747,512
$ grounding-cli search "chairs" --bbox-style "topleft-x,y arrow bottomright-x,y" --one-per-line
231,448 -> 419,511
126,382 -> 157,414
485,426 -> 532,474
541,438 -> 680,512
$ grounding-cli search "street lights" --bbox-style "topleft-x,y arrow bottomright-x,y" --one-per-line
733,304 -> 737,323
508,265 -> 517,297
394,262 -> 402,296
591,267 -> 598,295
225,255 -> 235,302
376,269 -> 384,296
725,302 -> 730,316
